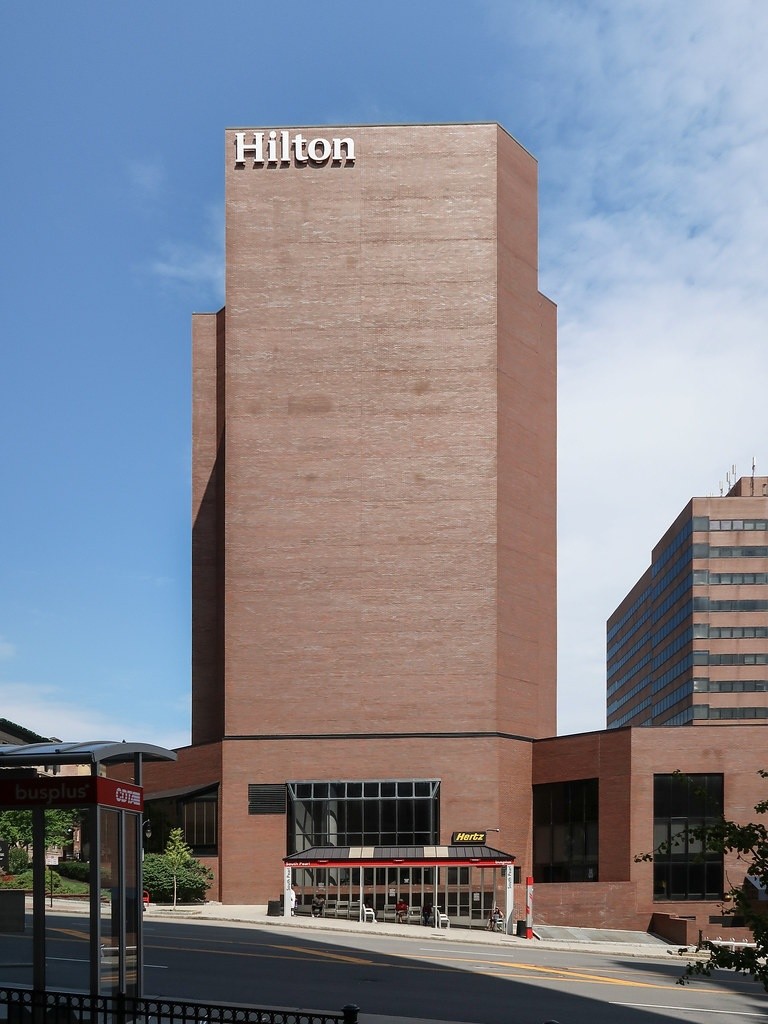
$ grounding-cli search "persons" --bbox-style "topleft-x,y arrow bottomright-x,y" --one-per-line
395,899 -> 408,923
291,884 -> 296,916
485,906 -> 504,931
312,894 -> 325,917
366,903 -> 378,922
422,903 -> 431,925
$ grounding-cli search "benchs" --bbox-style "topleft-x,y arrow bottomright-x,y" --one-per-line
384,905 -> 422,924
363,904 -> 375,922
437,911 -> 450,928
